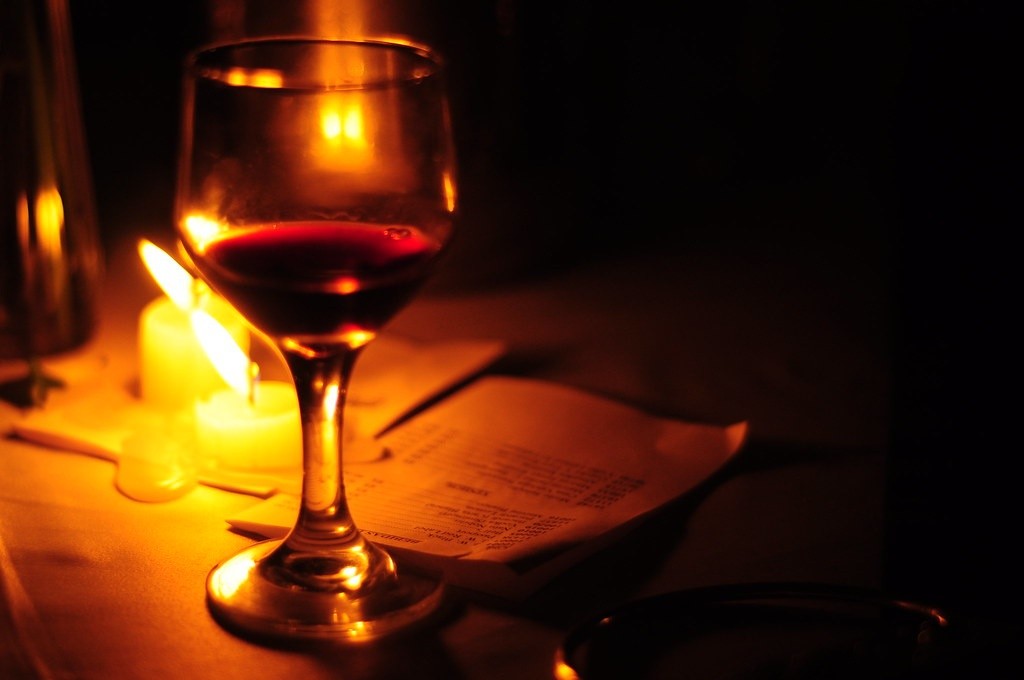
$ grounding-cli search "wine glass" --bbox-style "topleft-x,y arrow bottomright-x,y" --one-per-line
173,38 -> 460,656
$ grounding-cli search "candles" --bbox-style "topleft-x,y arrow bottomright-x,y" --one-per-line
140,283 -> 255,424
196,362 -> 306,472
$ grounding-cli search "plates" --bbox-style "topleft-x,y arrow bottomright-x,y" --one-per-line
551,575 -> 958,680
